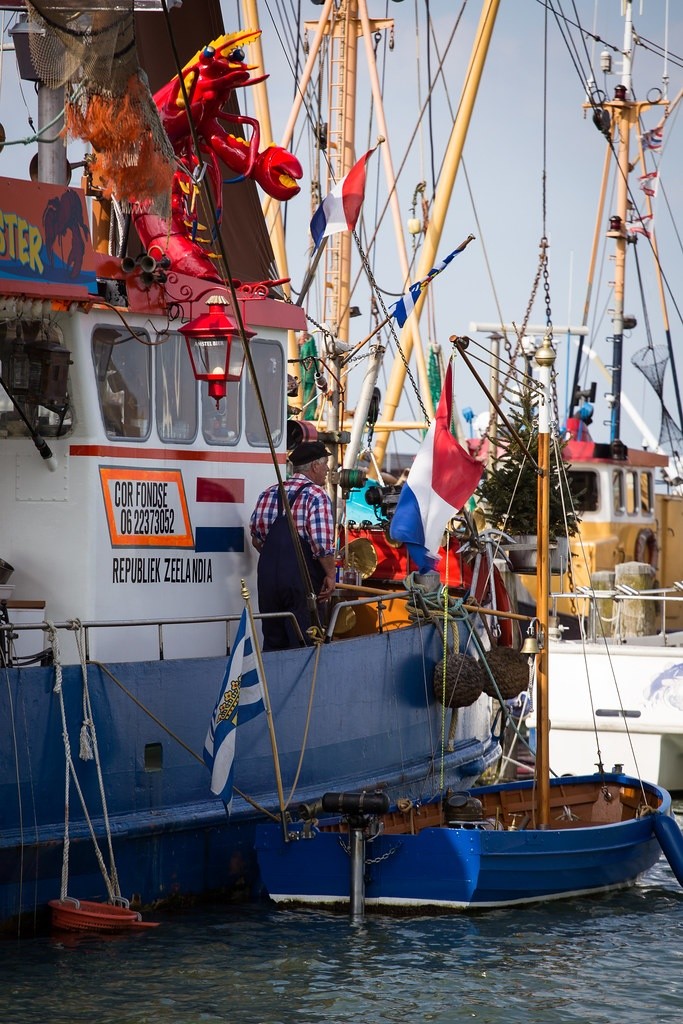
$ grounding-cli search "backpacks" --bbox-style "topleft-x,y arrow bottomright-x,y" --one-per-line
257,483 -> 327,616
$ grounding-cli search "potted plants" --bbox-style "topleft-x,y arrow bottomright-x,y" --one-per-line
472,365 -> 588,572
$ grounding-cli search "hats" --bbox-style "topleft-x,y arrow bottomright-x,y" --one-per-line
288,440 -> 332,466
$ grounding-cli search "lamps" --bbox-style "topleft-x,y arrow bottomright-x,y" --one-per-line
151,270 -> 269,411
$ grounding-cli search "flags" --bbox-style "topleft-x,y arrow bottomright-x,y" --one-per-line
310,146 -> 377,257
390,353 -> 487,575
203,606 -> 268,823
624,125 -> 664,239
388,245 -> 466,328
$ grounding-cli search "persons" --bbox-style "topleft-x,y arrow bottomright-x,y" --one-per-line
249,441 -> 337,653
567,404 -> 593,443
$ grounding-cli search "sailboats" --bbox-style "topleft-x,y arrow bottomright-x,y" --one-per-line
0,0 -> 683,929
